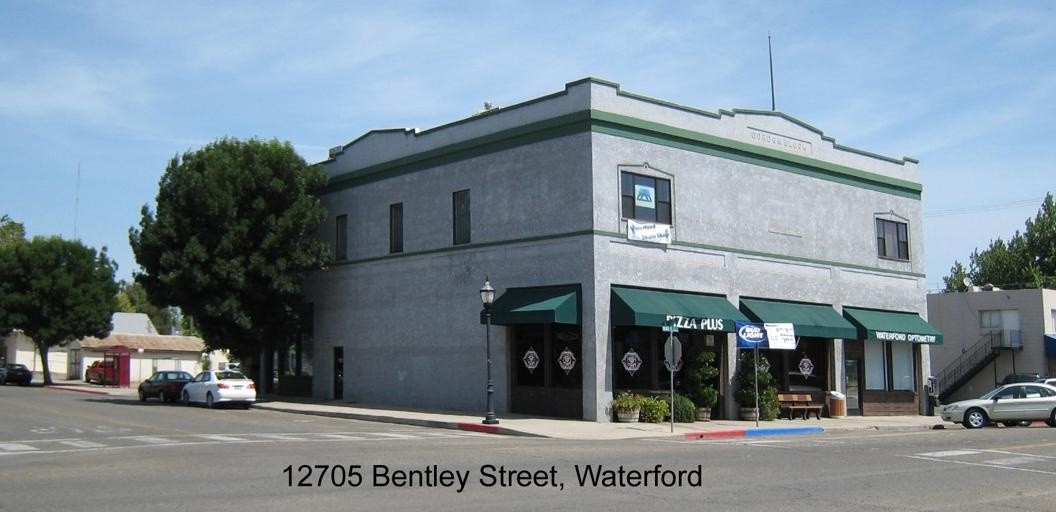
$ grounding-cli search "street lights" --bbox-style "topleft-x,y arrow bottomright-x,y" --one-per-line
480,275 -> 500,424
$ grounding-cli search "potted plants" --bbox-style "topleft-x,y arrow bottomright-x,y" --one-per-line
610,350 -> 781,422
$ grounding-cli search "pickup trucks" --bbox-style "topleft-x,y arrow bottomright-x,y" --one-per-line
86,360 -> 116,385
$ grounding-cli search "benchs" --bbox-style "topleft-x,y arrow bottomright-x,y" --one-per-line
778,394 -> 823,420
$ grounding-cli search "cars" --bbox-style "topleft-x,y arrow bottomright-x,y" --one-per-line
0,363 -> 33,386
138,369 -> 257,409
941,372 -> 1056,428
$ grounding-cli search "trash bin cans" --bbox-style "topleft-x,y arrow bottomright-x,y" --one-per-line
829,391 -> 847,419
